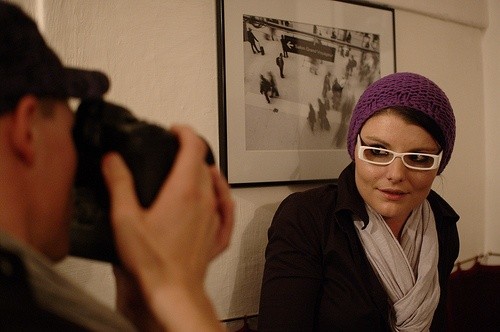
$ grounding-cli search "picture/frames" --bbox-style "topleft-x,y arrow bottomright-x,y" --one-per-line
216,0 -> 397,189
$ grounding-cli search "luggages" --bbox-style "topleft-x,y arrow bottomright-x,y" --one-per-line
258,41 -> 265,55
323,116 -> 330,130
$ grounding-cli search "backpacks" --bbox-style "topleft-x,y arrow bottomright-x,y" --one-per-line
276,57 -> 281,65
353,60 -> 357,67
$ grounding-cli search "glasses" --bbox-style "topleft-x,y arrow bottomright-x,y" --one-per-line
357,134 -> 443,170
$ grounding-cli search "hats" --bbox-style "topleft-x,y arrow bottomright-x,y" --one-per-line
0,0 -> 110,100
347,72 -> 456,176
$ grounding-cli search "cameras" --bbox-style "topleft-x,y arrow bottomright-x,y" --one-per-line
66,99 -> 215,264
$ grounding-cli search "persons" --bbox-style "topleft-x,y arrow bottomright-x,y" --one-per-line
257,72 -> 462,332
1,0 -> 236,332
243,14 -> 381,150
